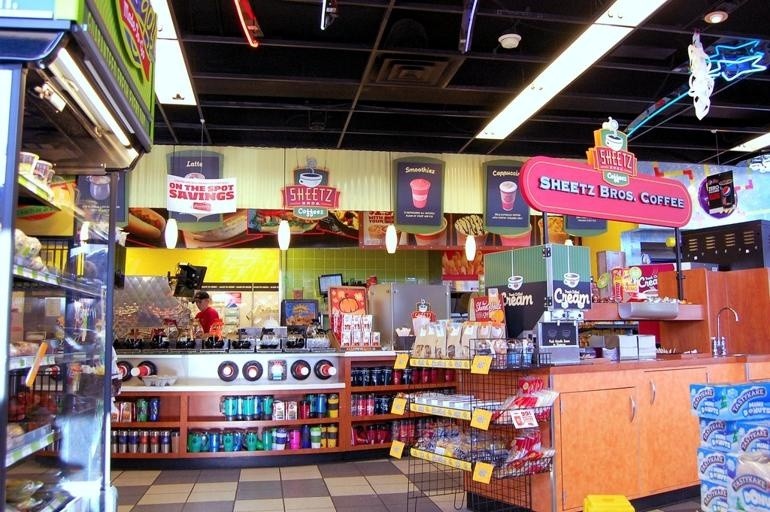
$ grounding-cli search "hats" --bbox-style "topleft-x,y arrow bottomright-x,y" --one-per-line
191,292 -> 209,303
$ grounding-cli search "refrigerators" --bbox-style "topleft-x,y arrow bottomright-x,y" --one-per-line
0,15 -> 155,512
368,282 -> 453,346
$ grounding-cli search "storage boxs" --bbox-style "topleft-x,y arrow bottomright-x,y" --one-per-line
688,383 -> 769,511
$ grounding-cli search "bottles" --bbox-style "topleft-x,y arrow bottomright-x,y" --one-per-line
65,367 -> 82,394
138,398 -> 159,421
261,423 -> 338,450
300,394 -> 340,418
110,431 -> 179,453
351,417 -> 459,444
351,390 -> 454,415
351,367 -> 454,386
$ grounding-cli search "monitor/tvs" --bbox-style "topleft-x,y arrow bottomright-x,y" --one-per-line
318,273 -> 343,295
172,262 -> 207,298
450,291 -> 473,314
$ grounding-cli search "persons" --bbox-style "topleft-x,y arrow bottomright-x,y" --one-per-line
193,293 -> 224,336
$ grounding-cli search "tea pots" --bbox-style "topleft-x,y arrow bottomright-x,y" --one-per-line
232,327 -> 252,348
124,328 -> 145,348
175,327 -> 201,348
261,327 -> 278,348
307,318 -> 330,349
287,326 -> 305,347
203,327 -> 224,348
150,327 -> 175,347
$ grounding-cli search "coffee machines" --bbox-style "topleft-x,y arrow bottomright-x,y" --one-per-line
483,241 -> 593,365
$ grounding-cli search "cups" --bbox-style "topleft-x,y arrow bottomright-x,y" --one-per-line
500,223 -> 534,246
474,346 -> 534,368
563,272 -> 581,288
143,374 -> 177,385
506,276 -> 523,290
538,216 -> 570,244
298,173 -> 322,187
223,364 -> 338,379
188,430 -> 257,452
414,217 -> 448,246
218,395 -> 273,418
606,134 -> 624,150
456,217 -> 488,246
720,179 -> 733,206
409,179 -> 431,209
45,363 -> 152,379
88,177 -> 111,201
499,182 -> 516,211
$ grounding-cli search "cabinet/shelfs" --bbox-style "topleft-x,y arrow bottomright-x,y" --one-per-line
398,342 -> 554,512
641,363 -> 770,492
456,370 -> 639,512
